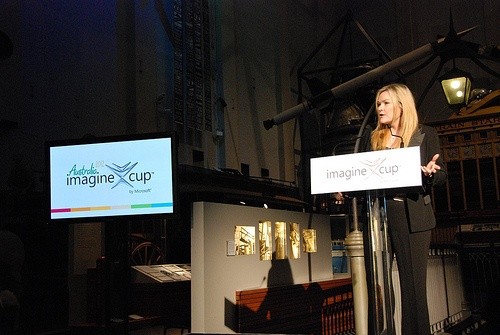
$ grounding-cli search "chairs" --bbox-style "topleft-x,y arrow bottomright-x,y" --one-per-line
95,256 -> 164,335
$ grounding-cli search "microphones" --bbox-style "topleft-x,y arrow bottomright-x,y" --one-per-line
387,123 -> 404,148
332,125 -> 371,156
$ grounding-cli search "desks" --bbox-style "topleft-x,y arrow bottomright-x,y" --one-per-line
127,264 -> 191,335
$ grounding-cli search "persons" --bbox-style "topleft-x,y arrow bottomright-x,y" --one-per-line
334,84 -> 448,335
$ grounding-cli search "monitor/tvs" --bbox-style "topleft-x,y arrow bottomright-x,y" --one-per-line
43,130 -> 180,225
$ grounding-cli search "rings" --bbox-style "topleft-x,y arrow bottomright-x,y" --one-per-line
428,173 -> 432,177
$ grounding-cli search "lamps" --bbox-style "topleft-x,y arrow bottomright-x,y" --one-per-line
437,60 -> 473,111
